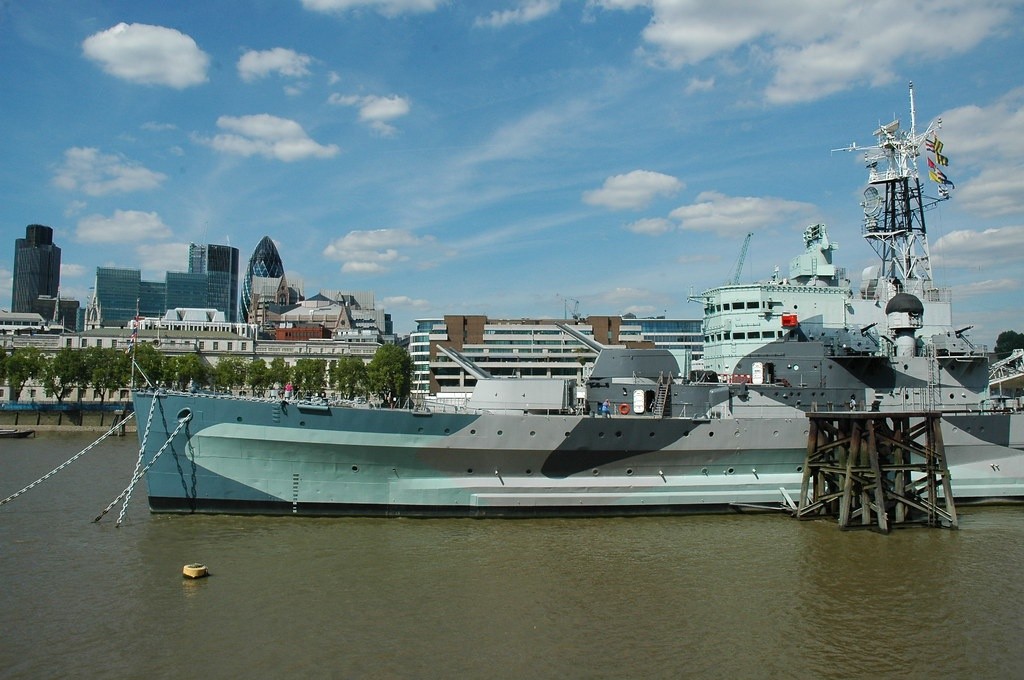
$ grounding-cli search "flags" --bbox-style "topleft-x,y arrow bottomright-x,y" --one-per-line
937,153 -> 948,165
934,137 -> 943,153
937,168 -> 954,189
928,170 -> 936,181
928,158 -> 936,170
926,140 -> 934,152
125,311 -> 139,353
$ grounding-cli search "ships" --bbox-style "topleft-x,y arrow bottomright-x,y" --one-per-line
130,80 -> 1024,522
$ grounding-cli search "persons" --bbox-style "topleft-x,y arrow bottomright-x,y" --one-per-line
602,400 -> 612,418
850,394 -> 857,412
284,382 -> 293,400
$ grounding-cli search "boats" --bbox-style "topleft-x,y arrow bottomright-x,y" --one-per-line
0,428 -> 35,438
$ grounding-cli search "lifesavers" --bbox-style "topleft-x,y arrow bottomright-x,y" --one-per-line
619,404 -> 630,414
